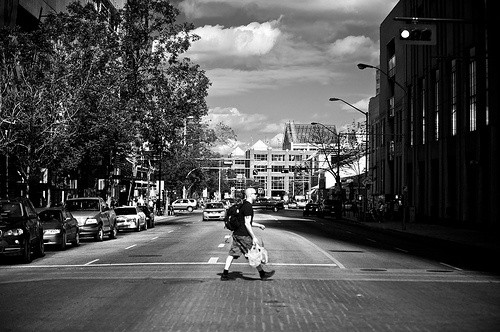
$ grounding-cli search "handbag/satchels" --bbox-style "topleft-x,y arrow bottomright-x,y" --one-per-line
260,247 -> 269,265
245,242 -> 262,268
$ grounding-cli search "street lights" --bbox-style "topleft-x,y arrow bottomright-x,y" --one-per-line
357,64 -> 410,230
311,122 -> 340,176
329,98 -> 368,180
184,116 -> 194,145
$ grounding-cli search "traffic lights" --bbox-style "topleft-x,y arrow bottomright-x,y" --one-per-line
398,24 -> 435,46
281,169 -> 289,173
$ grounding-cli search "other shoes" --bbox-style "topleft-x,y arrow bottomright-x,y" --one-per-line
221,275 -> 235,281
261,270 -> 275,281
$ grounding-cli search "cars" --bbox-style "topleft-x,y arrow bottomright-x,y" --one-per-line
203,200 -> 230,221
303,203 -> 321,217
1,198 -> 155,263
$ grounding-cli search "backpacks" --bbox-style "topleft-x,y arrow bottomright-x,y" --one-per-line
225,203 -> 244,231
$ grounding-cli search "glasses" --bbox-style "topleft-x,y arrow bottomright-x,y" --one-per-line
253,193 -> 257,195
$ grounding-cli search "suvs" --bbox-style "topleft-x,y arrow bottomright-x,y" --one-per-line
171,199 -> 197,212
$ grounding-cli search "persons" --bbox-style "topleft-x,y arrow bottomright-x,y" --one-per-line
220,187 -> 276,281
377,201 -> 386,223
109,197 -> 115,207
